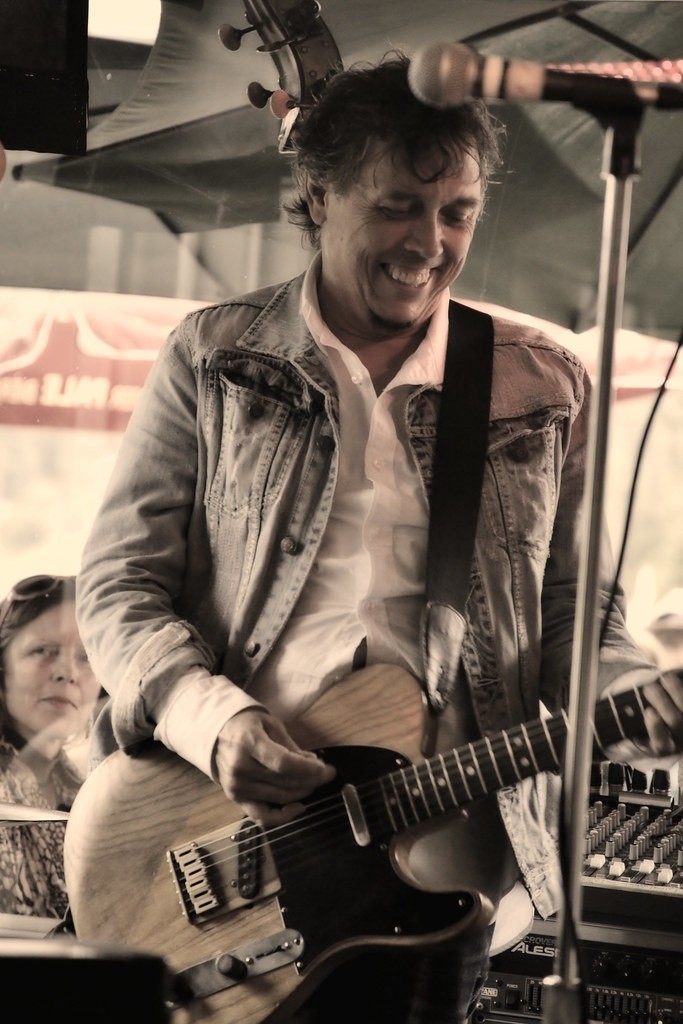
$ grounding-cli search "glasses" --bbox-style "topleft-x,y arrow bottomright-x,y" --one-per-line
0,574 -> 76,627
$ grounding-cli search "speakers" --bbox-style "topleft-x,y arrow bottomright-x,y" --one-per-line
0,0 -> 88,158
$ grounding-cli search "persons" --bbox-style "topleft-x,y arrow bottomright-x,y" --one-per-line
0,574 -> 102,934
74,58 -> 683,1024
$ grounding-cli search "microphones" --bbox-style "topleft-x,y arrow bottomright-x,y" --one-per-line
408,42 -> 683,111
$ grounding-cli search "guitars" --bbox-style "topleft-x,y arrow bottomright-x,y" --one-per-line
63,664 -> 683,1023
